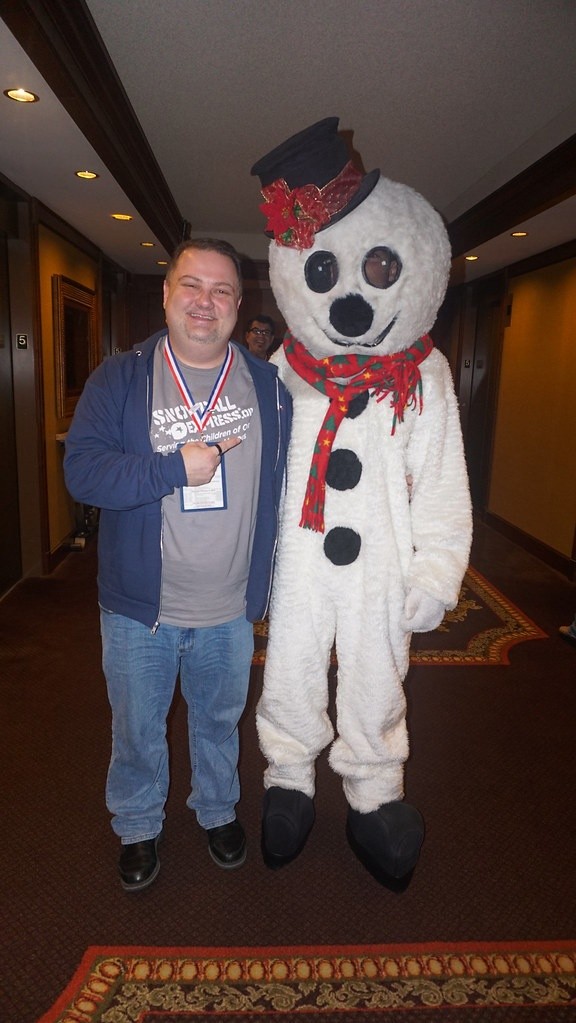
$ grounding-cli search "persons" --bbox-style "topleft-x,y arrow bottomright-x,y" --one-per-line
64,238 -> 292,892
249,115 -> 472,892
244,315 -> 276,362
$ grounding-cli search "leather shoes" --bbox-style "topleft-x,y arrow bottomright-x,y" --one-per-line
207,822 -> 247,869
119,836 -> 160,893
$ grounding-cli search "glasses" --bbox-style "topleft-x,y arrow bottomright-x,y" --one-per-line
251,329 -> 273,337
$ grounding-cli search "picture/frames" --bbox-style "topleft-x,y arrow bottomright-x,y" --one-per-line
53,272 -> 100,419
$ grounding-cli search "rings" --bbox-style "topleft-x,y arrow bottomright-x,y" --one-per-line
215,444 -> 222,455
